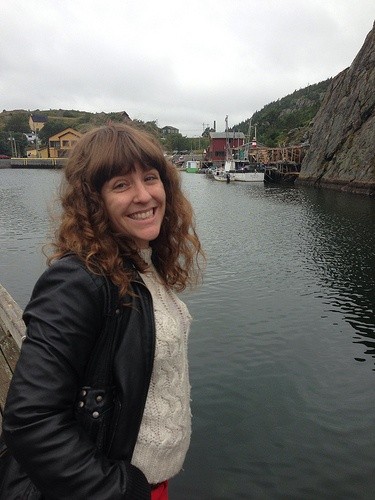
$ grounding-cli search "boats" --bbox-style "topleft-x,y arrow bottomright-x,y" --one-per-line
207,115 -> 265,184
263,167 -> 299,184
185,161 -> 211,174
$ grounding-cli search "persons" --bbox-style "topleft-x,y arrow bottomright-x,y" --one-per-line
0,122 -> 208,500
